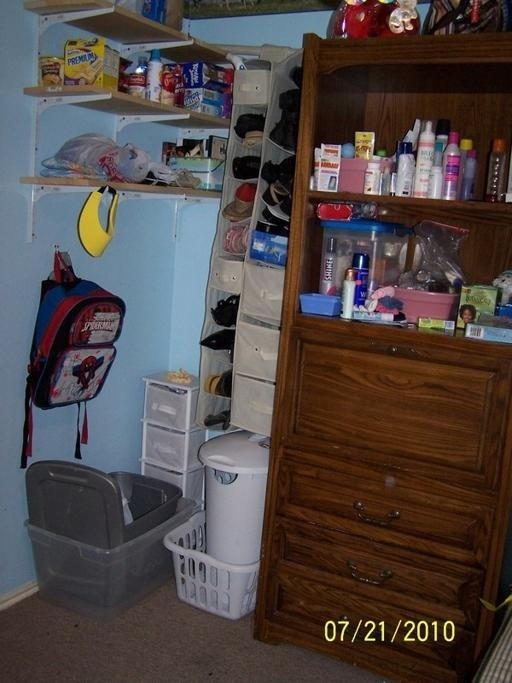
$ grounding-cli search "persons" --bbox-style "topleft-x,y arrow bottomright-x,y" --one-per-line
459,303 -> 477,325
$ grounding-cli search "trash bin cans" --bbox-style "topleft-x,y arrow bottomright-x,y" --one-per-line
197,428 -> 272,605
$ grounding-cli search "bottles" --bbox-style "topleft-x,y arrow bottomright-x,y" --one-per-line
486,136 -> 509,204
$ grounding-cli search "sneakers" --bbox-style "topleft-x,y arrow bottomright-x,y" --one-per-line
203,370 -> 232,397
212,293 -> 240,327
231,155 -> 261,180
233,113 -> 264,144
255,65 -> 302,240
203,409 -> 230,430
223,182 -> 257,223
221,226 -> 250,255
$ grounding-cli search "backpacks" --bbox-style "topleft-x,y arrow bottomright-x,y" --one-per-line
28,249 -> 126,411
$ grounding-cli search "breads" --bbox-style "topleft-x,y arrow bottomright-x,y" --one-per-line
168,372 -> 191,383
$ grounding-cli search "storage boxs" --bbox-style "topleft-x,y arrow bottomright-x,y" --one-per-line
23,461 -> 207,625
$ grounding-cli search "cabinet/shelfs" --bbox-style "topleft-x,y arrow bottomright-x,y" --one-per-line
252,35 -> 512,682
138,370 -> 207,510
21,0 -> 237,245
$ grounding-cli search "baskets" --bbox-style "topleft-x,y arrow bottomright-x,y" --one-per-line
162,509 -> 261,622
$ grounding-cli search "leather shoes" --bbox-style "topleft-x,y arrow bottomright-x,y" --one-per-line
199,328 -> 234,351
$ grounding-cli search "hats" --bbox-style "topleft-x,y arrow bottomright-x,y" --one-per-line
77,186 -> 120,259
